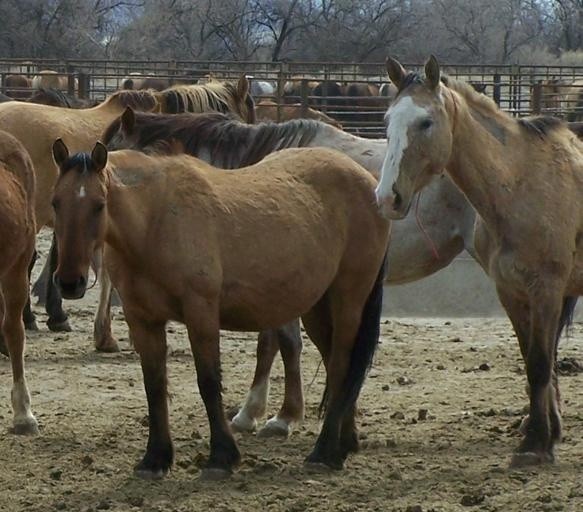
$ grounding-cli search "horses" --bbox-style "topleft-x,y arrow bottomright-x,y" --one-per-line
0,53 -> 583,482
